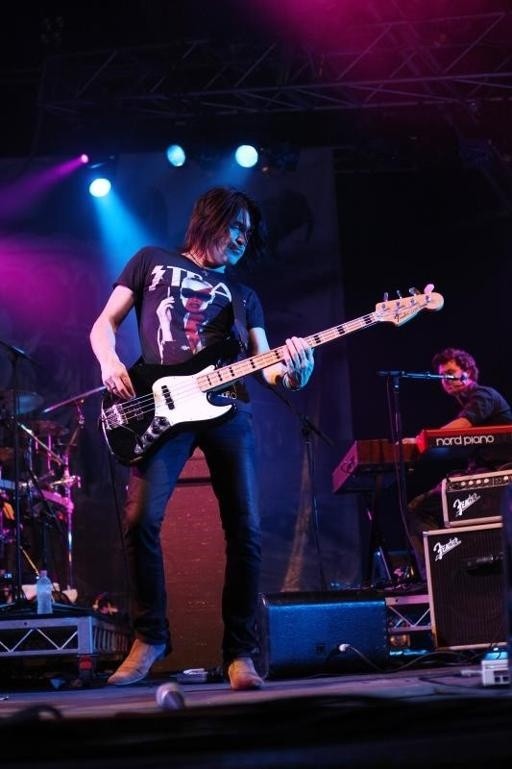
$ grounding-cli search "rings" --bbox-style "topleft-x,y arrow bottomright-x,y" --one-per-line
111,384 -> 116,389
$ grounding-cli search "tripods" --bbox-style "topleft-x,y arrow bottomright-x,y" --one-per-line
0,360 -> 90,611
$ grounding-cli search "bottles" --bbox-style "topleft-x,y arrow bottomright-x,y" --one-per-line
36,570 -> 54,615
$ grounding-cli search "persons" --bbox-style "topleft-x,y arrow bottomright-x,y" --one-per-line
89,187 -> 315,691
384,348 -> 512,594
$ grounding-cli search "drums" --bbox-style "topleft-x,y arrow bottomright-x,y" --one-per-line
0,425 -> 29,492
15,486 -> 73,543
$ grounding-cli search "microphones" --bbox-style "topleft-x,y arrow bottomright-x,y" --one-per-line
443,373 -> 473,384
157,682 -> 185,710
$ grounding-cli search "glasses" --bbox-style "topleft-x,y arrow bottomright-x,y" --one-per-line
179,288 -> 211,301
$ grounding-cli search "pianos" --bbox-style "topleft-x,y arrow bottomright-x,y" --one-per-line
331,437 -> 416,492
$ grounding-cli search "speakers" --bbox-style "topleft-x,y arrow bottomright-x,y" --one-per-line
254,591 -> 392,678
427,526 -> 512,651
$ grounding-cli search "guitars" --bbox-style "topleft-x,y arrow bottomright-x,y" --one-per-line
99,283 -> 444,468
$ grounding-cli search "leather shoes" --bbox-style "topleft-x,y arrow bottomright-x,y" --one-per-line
228,657 -> 264,689
106,638 -> 166,685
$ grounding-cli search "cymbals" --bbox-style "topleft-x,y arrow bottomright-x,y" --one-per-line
0,389 -> 45,418
16,420 -> 71,442
